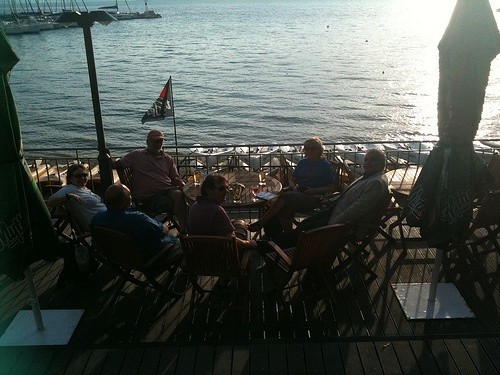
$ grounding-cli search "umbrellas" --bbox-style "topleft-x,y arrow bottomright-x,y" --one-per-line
0,24 -> 74,331
403,0 -> 500,300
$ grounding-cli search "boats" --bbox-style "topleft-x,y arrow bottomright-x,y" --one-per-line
28,137 -> 500,196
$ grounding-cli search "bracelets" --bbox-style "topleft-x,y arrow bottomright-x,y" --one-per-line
66,194 -> 70,199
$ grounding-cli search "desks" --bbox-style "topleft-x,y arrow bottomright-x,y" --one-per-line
183,171 -> 282,241
385,166 -> 423,234
391,189 -> 410,197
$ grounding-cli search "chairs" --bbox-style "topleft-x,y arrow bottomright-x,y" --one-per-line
44,156 -> 500,307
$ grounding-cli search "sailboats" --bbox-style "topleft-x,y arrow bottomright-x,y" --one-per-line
0,0 -> 164,35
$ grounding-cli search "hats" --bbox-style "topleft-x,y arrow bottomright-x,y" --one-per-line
147,130 -> 165,140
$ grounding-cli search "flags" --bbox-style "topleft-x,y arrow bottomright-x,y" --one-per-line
142,75 -> 174,124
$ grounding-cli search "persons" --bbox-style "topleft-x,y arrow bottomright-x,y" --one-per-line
276,149 -> 389,249
46,163 -> 107,231
187,174 -> 258,266
112,130 -> 186,235
248,138 -> 338,232
90,185 -> 184,267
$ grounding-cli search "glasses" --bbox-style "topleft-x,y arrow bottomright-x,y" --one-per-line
72,173 -> 88,178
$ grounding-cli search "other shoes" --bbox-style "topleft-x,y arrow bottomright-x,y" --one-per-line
248,222 -> 262,232
